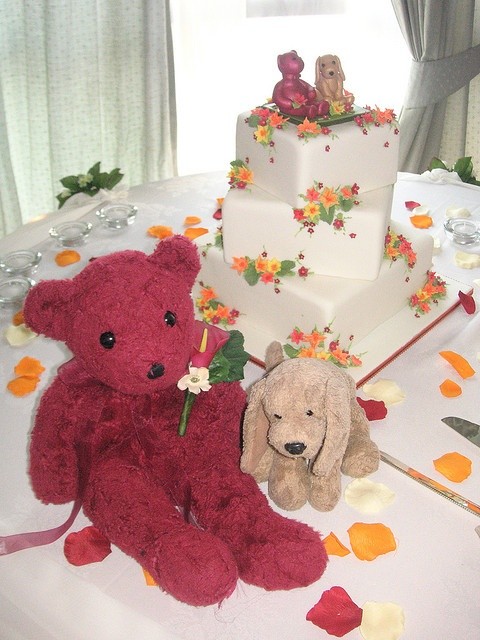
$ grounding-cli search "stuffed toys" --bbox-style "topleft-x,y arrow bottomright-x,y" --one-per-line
241,341 -> 379,510
0,233 -> 328,605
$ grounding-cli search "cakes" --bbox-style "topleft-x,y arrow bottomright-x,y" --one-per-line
201,101 -> 433,354
